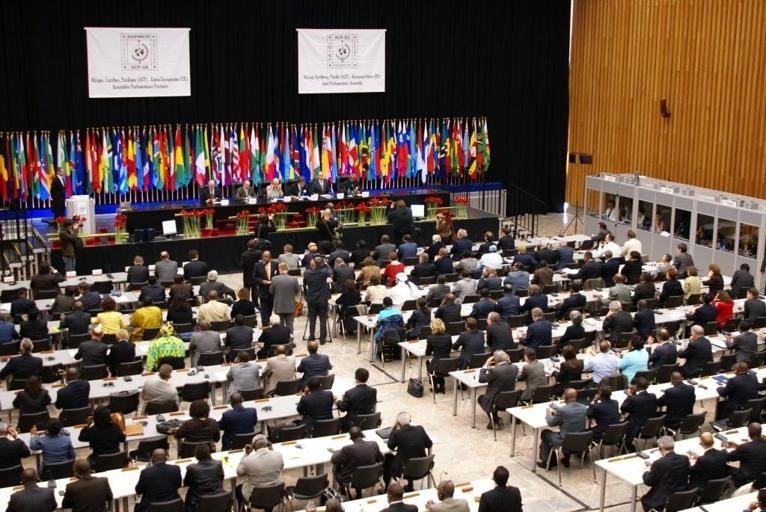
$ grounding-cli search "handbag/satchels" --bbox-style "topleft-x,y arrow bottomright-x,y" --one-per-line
407,378 -> 423,398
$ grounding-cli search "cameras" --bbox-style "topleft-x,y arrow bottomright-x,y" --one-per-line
77,222 -> 84,228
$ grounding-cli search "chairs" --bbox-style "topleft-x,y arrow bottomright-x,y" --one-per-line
663,410 -> 708,440
0,464 -> 24,488
649,487 -> 701,512
673,488 -> 766,512
180,440 -> 215,458
287,177 -> 305,195
537,344 -> 558,356
624,414 -> 668,451
354,411 -> 382,430
229,348 -> 256,360
702,321 -> 720,334
199,179 -> 225,200
267,423 -> 309,443
336,174 -> 357,193
286,268 -> 302,273
420,325 -> 433,339
724,317 -> 741,330
80,363 -> 109,379
446,320 -> 466,334
237,482 -> 285,512
746,396 -> 766,419
274,378 -> 305,397
388,453 -> 436,491
699,475 -> 733,502
229,431 -> 263,449
182,379 -> 212,403
643,298 -> 658,309
508,314 -> 528,327
193,489 -> 234,512
314,417 -> 343,437
338,461 -> 385,499
503,348 -> 526,361
469,350 -> 493,368
258,180 -> 284,195
117,359 -> 143,375
130,436 -> 169,462
313,177 -> 332,194
285,472 -> 337,511
615,327 -> 639,346
585,421 -> 628,461
142,497 -> 184,511
160,356 -> 185,368
230,181 -> 255,197
235,387 -> 271,401
307,373 -> 335,390
546,429 -> 597,490
197,351 -> 224,365
97,450 -> 128,471
567,337 -> 585,351
711,407 -> 753,431
665,295 -> 681,307
40,459 -> 74,481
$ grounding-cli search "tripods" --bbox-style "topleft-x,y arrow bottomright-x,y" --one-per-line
558,164 -> 585,237
302,313 -> 333,344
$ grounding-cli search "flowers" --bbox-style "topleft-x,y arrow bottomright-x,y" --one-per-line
305,206 -> 322,227
425,196 -> 443,217
367,198 -> 389,222
233,210 -> 250,235
201,209 -> 216,231
179,208 -> 204,239
354,202 -> 368,225
256,202 -> 289,230
453,197 -> 469,219
112,214 -> 130,245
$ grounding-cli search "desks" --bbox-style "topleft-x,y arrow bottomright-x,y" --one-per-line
505,363 -> 766,472
450,324 -> 766,428
594,422 -> 766,512
117,188 -> 451,232
0,349 -> 329,411
349,234 -> 626,269
0,423 -> 416,512
5,261 -> 270,347
351,270 -> 735,361
397,290 -> 766,383
293,481 -> 510,512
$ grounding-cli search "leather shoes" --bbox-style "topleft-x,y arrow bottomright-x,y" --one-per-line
487,418 -> 505,431
537,461 -> 547,468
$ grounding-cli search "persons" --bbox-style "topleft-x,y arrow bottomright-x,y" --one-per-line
218,393 -> 258,451
269,262 -> 301,333
374,297 -> 405,360
715,362 -> 759,420
436,209 -> 453,245
187,320 -> 221,367
231,288 -> 255,322
577,385 -> 621,460
383,251 -> 404,287
602,300 -> 634,347
485,312 -> 519,363
427,274 -> 450,307
727,422 -> 766,488
242,238 -> 261,307
553,310 -> 586,355
167,295 -> 193,325
107,329 -> 140,378
135,449 -> 183,512
322,498 -> 346,512
127,256 -> 150,290
434,248 -> 454,281
30,418 -> 77,481
696,227 -> 705,239
679,293 -> 718,339
336,279 -> 362,336
332,257 -> 355,282
555,282 -> 586,320
0,309 -> 21,356
479,245 -> 503,273
520,284 -> 548,326
378,484 -> 418,512
0,337 -> 44,391
536,388 -> 588,470
258,314 -> 290,359
174,399 -> 220,459
469,288 -> 497,321
621,230 -> 641,262
594,221 -> 615,249
142,324 -> 185,375
278,244 -> 302,274
478,350 -> 519,430
477,466 -> 523,512
454,249 -> 480,279
356,256 -> 380,289
60,301 -> 91,348
631,299 -> 656,341
386,200 -> 415,244
407,298 -> 431,341
653,254 -> 673,280
424,234 -> 446,263
682,265 -> 703,304
519,307 -> 553,359
512,244 -> 539,273
236,434 -> 285,512
139,276 -> 165,307
50,286 -> 75,321
673,243 -> 694,274
389,272 -> 411,305
504,263 -> 529,295
141,363 -> 181,415
0,421 -> 30,488
372,234 -> 396,267
74,328 -> 107,380
349,240 -> 370,268
638,210 -> 645,228
597,233 -> 621,259
738,287 -> 766,327
454,269 -> 477,304
533,239 -> 559,271
183,444 -> 225,511
62,459 -> 113,512
690,431 -> 729,495
621,251 -> 642,284
303,256 -> 332,345
20,309 -> 52,353
648,326 -> 679,384
335,368 -> 378,433
329,240 -> 349,268
59,218 -> 84,271
658,268 -> 684,308
154,251 -> 178,287
609,273 -> 633,313
475,231 -> 500,259
302,242 -> 320,269
640,435 -> 689,512
743,488 -> 766,512
567,252 -> 602,284
199,290 -> 231,323
656,372 -> 696,436
712,290 -> 734,332
425,480 -> 470,512
97,297 -> 125,344
263,345 -> 297,397
377,413 -> 433,494
703,263 -> 724,298
451,228 -> 473,261
531,260 -> 553,293
425,318 -> 452,394
600,251 -> 619,287
253,251 -> 278,326
434,293 -> 462,335
497,228 -> 515,257
725,321 -> 758,363
296,342 -> 332,391
170,273 -> 194,298
226,351 -> 263,398
621,206 -> 632,224
11,287 -> 36,324
497,283 -> 521,319
12,376 -> 51,433
297,378 -> 334,438
452,317 -> 486,390
226,314 -> 254,361
128,296 -> 163,341
183,249 -> 208,279
730,262 -> 755,299
365,275 -> 387,335
477,267 -> 502,301
618,336 -> 649,388
511,347 -> 548,424
409,253 -> 435,285
655,214 -> 664,234
677,325 -> 714,379
605,199 -> 616,221
199,172 -> 367,237
584,341 -> 619,389
633,272 -> 656,311
332,426 -> 386,498
621,376 -> 659,452
56,367 -> 91,426
5,468 -> 57,512
78,406 -> 130,472
397,234 -> 417,262
557,239 -> 573,270
200,270 -> 237,301
550,347 -> 584,399
30,262 -> 66,300
77,282 -> 101,311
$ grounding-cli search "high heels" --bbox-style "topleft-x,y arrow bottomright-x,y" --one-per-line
430,387 -> 438,394
440,385 -> 446,393
